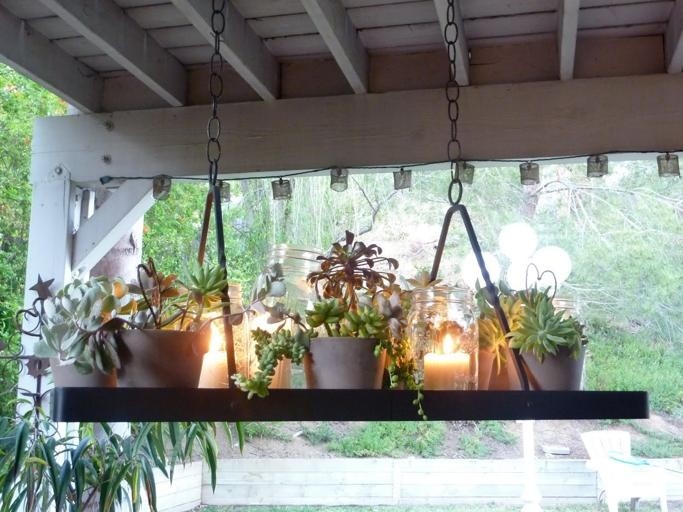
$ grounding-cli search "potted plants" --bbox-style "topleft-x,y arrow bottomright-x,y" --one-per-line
475,316 -> 507,392
473,277 -> 589,393
33,230 -> 288,397
228,231 -> 430,424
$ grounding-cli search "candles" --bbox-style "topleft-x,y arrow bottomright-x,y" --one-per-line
423,331 -> 473,392
198,326 -> 231,388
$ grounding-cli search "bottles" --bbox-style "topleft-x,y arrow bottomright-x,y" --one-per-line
409,289 -> 479,390
198,242 -> 325,391
